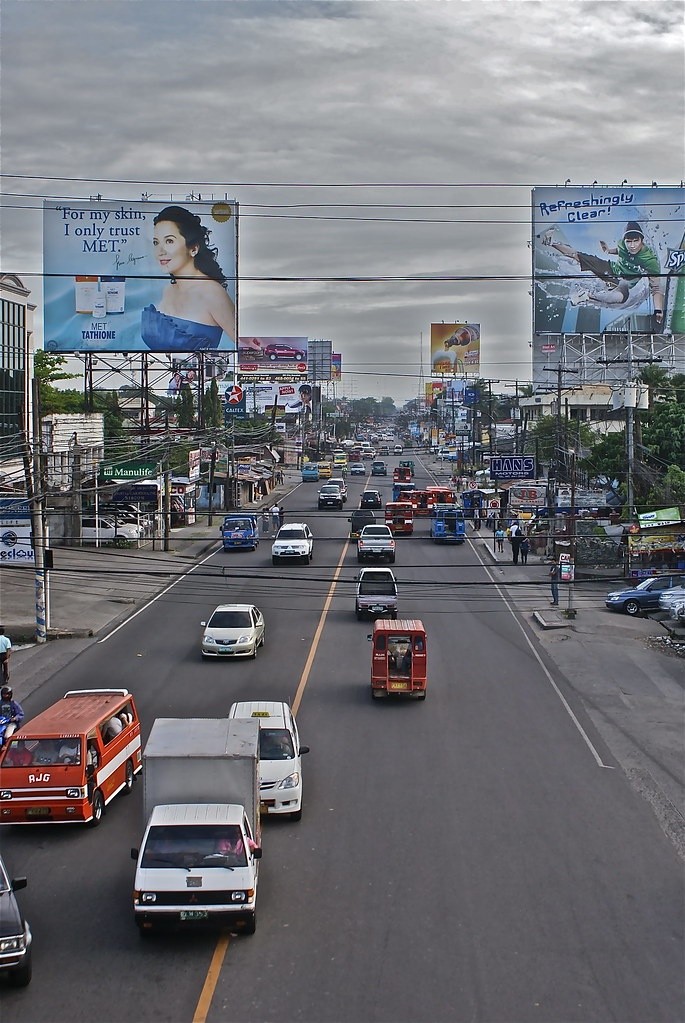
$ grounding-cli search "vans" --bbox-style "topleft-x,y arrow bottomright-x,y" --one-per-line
227,700 -> 310,820
0,688 -> 143,827
353,567 -> 399,620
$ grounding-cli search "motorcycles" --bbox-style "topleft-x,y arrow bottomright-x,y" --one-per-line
367,619 -> 428,700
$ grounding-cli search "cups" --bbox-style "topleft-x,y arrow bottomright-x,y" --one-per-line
433,351 -> 464,373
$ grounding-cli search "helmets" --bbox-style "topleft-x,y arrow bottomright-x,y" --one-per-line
1,688 -> 12,701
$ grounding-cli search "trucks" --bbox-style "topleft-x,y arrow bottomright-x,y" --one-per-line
220,513 -> 260,551
131,717 -> 263,938
347,510 -> 376,544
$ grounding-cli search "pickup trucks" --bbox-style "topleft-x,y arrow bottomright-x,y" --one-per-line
357,524 -> 396,564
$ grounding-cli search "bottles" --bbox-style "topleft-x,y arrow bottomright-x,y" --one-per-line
444,324 -> 479,347
92,293 -> 106,319
663,231 -> 685,333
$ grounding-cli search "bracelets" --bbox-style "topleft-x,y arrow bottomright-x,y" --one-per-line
654,309 -> 663,314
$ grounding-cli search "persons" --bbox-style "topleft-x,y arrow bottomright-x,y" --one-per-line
273,730 -> 290,753
60,737 -> 94,775
469,480 -> 476,489
472,501 -> 480,531
217,826 -> 258,857
1,740 -> 32,766
542,222 -> 664,324
549,558 -> 558,605
32,738 -> 58,765
401,636 -> 412,671
288,385 -> 312,412
262,503 -> 287,533
495,527 -> 505,553
140,206 -> 235,349
508,522 -> 529,564
100,710 -> 133,745
0,686 -> 24,740
342,465 -> 347,477
0,628 -> 12,685
452,475 -> 462,491
170,370 -> 196,394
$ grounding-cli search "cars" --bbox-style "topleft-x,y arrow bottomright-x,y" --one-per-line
657,583 -> 685,627
605,576 -> 685,614
300,426 -> 466,544
0,849 -> 35,987
200,603 -> 265,662
80,495 -> 186,548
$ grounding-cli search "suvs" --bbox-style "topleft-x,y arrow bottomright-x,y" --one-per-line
263,343 -> 306,361
272,523 -> 313,565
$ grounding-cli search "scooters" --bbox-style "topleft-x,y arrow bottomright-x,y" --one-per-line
0,716 -> 20,747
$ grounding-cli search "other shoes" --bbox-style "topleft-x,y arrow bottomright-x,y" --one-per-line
550,602 -> 558,605
